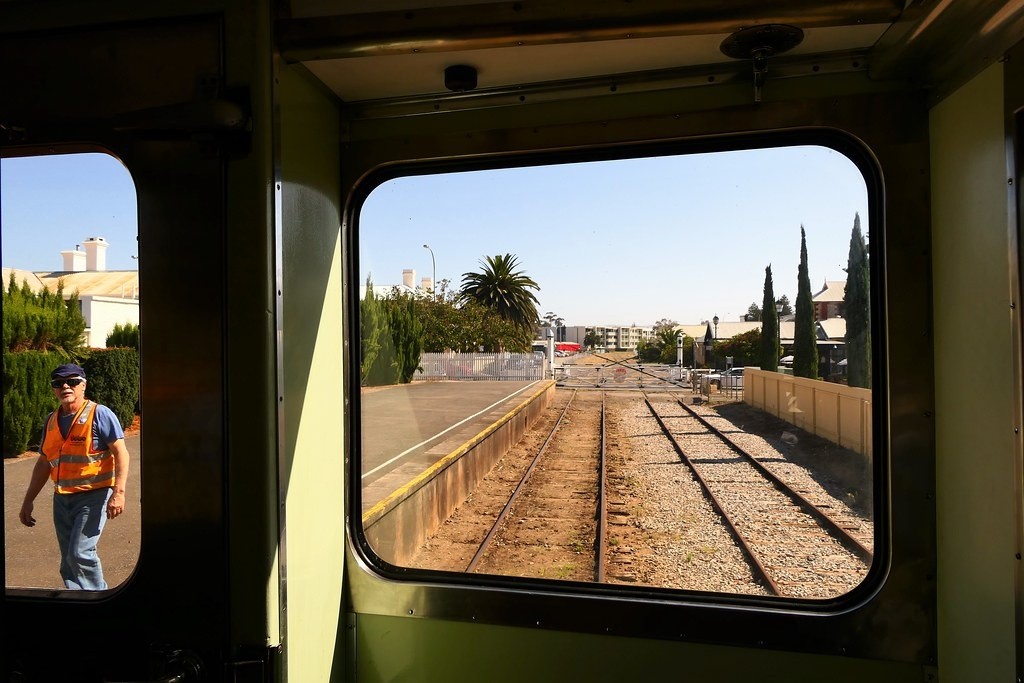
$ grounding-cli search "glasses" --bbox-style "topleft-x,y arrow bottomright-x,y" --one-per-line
51,379 -> 85,388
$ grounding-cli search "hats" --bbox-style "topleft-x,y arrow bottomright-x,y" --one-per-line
51,365 -> 86,379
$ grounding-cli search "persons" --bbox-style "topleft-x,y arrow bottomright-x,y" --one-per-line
19,364 -> 129,590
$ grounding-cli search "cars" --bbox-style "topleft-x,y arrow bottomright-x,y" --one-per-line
778,355 -> 795,372
705,367 -> 744,390
423,347 -> 570,379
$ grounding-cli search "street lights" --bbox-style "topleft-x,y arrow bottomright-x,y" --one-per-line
713,315 -> 719,340
423,245 -> 435,302
